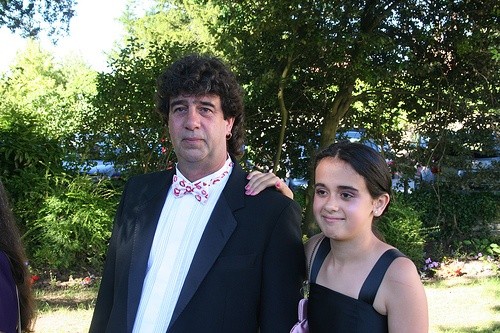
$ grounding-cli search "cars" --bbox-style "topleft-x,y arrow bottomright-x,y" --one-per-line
413,133 -> 500,191
289,130 -> 391,192
60,130 -> 254,182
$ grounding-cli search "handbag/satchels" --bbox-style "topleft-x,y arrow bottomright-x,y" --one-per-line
289,297 -> 309,333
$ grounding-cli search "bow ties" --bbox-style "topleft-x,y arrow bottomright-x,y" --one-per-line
172,162 -> 236,203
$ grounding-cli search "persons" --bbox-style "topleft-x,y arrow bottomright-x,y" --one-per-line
0,177 -> 40,333
245,142 -> 430,333
88,55 -> 307,333
